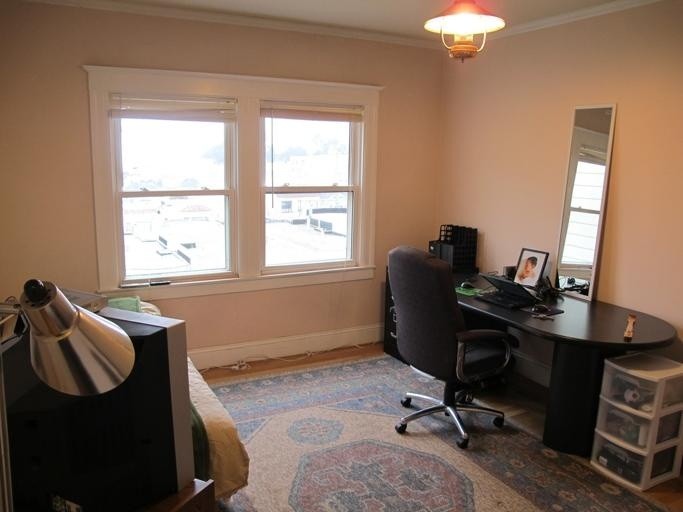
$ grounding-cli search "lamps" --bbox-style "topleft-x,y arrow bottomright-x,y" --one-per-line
2,275 -> 138,403
424,1 -> 507,64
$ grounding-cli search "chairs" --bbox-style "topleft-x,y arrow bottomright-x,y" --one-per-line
386,246 -> 520,450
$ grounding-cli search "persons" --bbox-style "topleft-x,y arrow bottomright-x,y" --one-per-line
518,256 -> 538,285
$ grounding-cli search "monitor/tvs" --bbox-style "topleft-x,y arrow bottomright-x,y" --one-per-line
0,305 -> 195,512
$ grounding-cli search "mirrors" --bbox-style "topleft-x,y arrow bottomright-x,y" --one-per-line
551,103 -> 616,302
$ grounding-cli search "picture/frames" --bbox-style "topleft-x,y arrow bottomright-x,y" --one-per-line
513,248 -> 548,287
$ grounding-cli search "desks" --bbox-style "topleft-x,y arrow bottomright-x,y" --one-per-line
456,272 -> 676,454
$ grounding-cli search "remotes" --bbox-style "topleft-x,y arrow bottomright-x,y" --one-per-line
121,283 -> 150,288
150,281 -> 170,286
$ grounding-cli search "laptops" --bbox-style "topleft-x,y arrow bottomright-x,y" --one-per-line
477,273 -> 540,310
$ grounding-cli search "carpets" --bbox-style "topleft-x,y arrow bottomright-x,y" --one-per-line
210,354 -> 673,511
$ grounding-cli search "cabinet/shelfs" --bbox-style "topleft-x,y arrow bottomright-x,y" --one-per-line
383,263 -> 411,363
588,355 -> 683,491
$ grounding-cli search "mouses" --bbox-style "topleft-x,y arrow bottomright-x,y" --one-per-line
532,304 -> 548,313
568,277 -> 575,284
461,282 -> 473,288
